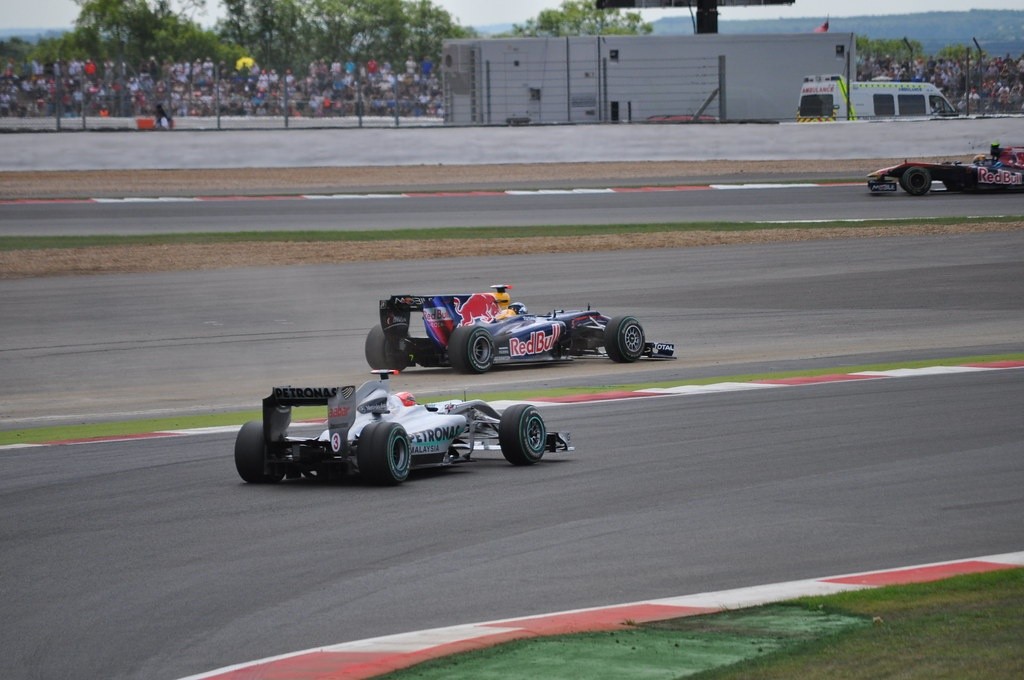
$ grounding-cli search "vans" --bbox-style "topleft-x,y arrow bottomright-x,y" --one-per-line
798,73 -> 958,123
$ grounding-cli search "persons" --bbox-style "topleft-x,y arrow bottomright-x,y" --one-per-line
0,48 -> 446,132
854,52 -> 1023,115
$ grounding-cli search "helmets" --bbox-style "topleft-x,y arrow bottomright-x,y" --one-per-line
972,155 -> 985,162
508,301 -> 528,316
395,391 -> 416,406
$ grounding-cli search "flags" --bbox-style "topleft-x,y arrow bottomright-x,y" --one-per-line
814,22 -> 828,32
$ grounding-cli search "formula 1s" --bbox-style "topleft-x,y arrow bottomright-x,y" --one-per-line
234,368 -> 578,489
363,282 -> 679,376
864,143 -> 1024,197
